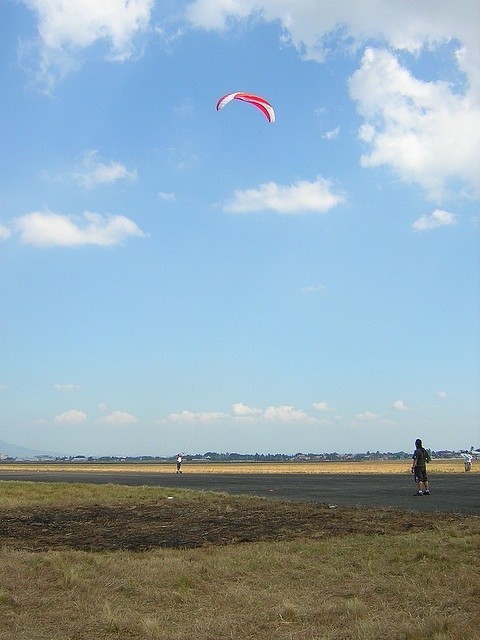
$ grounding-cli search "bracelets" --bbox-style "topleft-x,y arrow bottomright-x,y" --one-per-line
412,467 -> 415,469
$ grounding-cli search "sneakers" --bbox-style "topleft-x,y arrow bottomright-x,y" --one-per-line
413,491 -> 423,496
423,490 -> 431,495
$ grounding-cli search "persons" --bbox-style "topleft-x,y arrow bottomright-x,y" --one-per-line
175,453 -> 182,474
462,450 -> 473,473
411,439 -> 432,497
